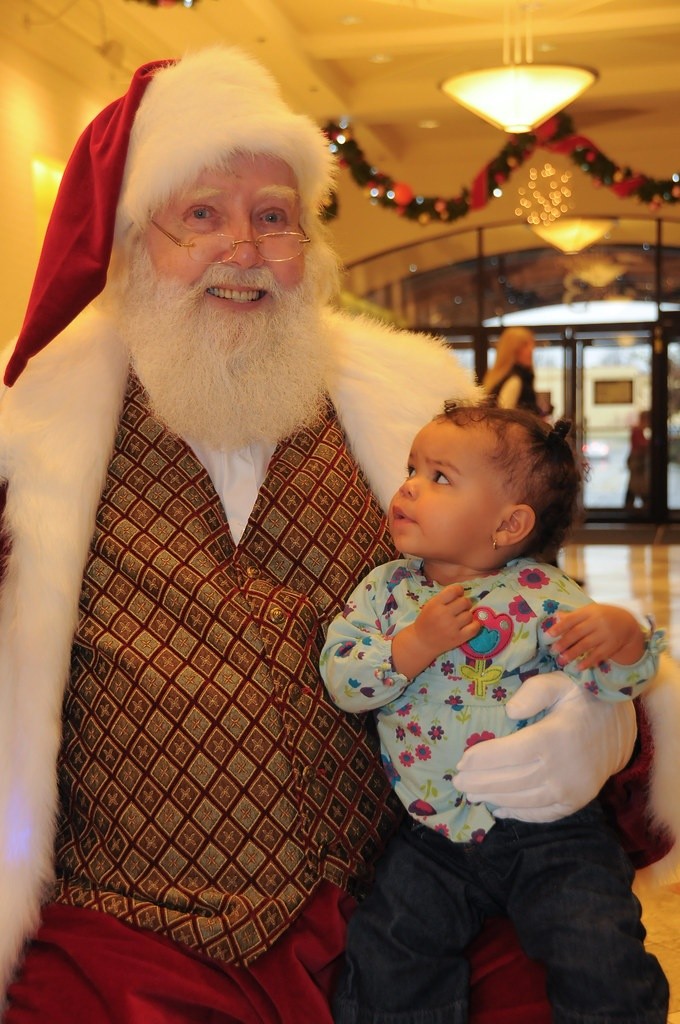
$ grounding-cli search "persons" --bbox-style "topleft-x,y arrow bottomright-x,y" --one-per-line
0,46 -> 680,1024
482,326 -> 586,587
318,405 -> 671,1024
624,410 -> 653,511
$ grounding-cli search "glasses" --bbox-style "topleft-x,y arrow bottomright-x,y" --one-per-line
147,216 -> 312,264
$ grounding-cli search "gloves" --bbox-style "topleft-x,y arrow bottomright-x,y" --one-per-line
454,668 -> 639,826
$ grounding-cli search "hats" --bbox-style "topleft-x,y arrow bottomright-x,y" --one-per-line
0,48 -> 336,403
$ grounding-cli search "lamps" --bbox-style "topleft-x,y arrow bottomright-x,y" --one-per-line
441,0 -> 597,135
525,214 -> 626,287
